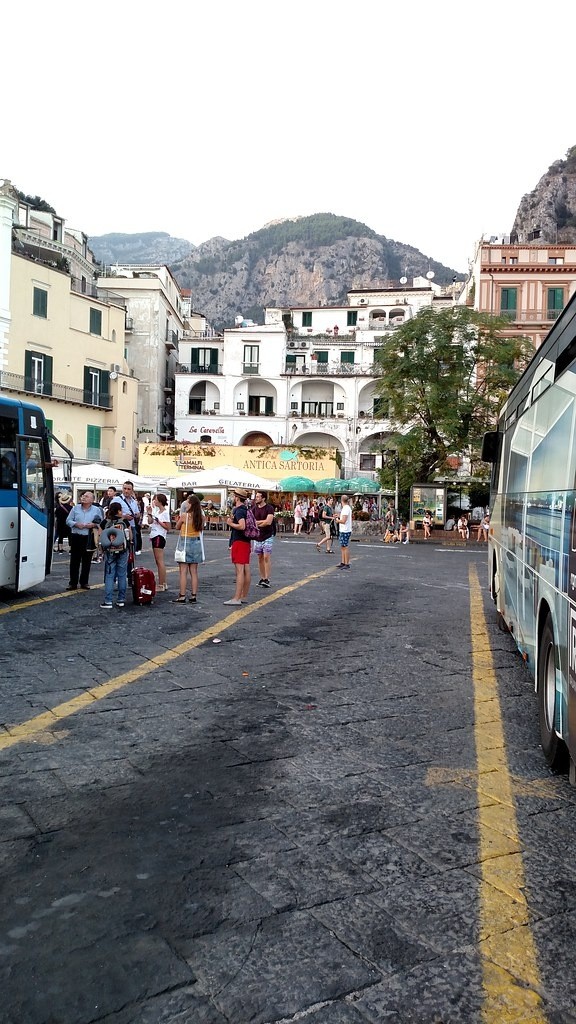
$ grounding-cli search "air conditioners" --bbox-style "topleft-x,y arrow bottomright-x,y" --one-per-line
358,297 -> 369,305
287,341 -> 299,349
359,410 -> 367,417
299,341 -> 310,349
110,363 -> 121,374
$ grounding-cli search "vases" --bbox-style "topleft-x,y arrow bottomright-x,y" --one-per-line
173,515 -> 294,523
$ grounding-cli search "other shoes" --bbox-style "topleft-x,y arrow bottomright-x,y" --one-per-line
223,599 -> 242,605
81,584 -> 90,589
68,550 -> 72,556
256,579 -> 264,588
340,565 -> 351,570
115,601 -> 125,607
336,563 -> 345,568
59,550 -> 63,555
241,598 -> 248,602
90,557 -> 101,564
155,583 -> 168,592
100,604 -> 113,609
261,579 -> 270,587
66,585 -> 77,590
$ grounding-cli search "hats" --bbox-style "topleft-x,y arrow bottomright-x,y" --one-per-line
231,489 -> 248,499
59,494 -> 71,503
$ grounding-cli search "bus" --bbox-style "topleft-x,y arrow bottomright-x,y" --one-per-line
0,396 -> 74,593
481,291 -> 576,788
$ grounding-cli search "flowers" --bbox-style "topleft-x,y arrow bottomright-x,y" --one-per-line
170,509 -> 294,516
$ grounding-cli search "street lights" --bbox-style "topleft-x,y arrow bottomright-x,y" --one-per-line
383,444 -> 398,518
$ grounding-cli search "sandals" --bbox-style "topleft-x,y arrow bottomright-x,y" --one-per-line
316,544 -> 321,552
326,549 -> 335,553
189,593 -> 196,604
169,593 -> 186,605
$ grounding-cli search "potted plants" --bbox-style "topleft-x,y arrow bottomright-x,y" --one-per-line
284,321 -> 294,333
353,511 -> 370,521
209,409 -> 216,415
290,412 -> 344,418
239,410 -> 275,416
203,408 -> 209,415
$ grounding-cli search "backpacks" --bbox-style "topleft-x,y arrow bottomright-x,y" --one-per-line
105,517 -> 126,554
318,508 -> 323,519
244,509 -> 260,538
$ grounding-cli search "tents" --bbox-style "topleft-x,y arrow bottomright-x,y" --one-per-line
25,463 -> 160,498
167,465 -> 281,516
281,477 -> 381,498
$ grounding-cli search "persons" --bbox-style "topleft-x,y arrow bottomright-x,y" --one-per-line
334,495 -> 352,570
100,481 -> 151,587
55,491 -> 103,590
223,488 -> 251,605
423,511 -> 433,539
316,497 -> 335,554
446,515 -> 468,541
147,494 -> 171,591
101,503 -> 132,608
293,497 -> 378,535
251,491 -> 274,588
384,497 -> 410,544
170,491 -> 205,604
2,447 -> 59,496
477,515 -> 489,544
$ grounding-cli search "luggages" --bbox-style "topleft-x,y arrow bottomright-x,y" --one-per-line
130,542 -> 156,605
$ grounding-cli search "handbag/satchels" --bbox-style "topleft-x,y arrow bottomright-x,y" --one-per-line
174,549 -> 187,562
